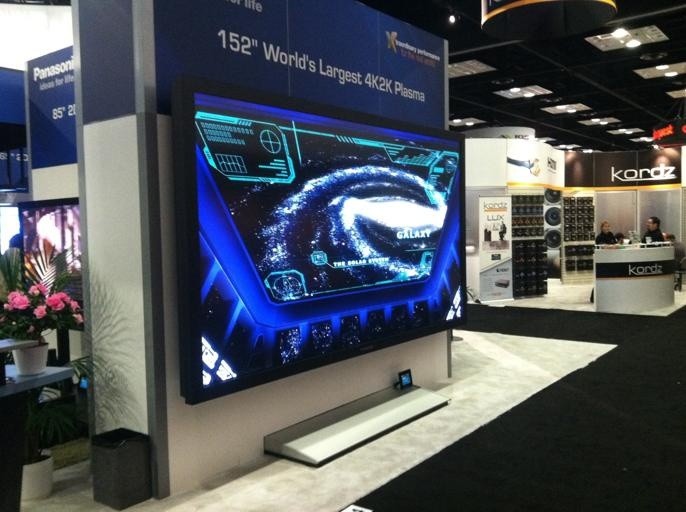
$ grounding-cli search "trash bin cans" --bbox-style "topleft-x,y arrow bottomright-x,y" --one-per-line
92,428 -> 152,510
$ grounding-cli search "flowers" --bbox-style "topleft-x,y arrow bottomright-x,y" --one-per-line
0,244 -> 84,342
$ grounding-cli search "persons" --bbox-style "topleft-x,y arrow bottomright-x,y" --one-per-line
615,233 -> 624,244
642,216 -> 666,248
596,220 -> 617,249
499,221 -> 506,241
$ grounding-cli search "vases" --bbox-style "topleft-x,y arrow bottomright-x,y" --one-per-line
11,342 -> 48,376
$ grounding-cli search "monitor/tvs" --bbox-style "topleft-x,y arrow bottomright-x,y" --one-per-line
17,197 -> 82,300
171,74 -> 468,405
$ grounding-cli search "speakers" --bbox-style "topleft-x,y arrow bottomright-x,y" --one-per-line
544,187 -> 562,278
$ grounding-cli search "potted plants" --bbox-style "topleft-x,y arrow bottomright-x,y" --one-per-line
20,356 -> 97,502
